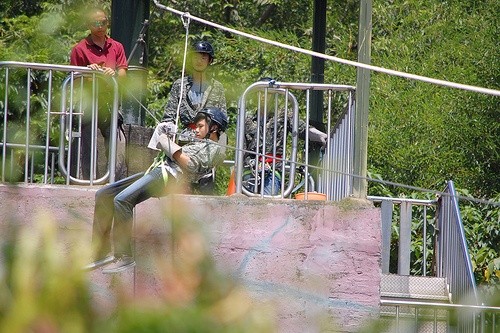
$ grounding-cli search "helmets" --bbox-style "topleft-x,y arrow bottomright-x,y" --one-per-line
196,106 -> 228,132
186,40 -> 217,60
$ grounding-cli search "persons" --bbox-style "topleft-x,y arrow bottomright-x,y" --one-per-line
242,77 -> 327,195
161,40 -> 227,195
81,106 -> 227,274
70,8 -> 127,186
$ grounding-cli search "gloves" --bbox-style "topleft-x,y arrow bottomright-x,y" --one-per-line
155,132 -> 183,162
145,123 -> 180,152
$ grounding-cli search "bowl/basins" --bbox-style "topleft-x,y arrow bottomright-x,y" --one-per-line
295,192 -> 326,200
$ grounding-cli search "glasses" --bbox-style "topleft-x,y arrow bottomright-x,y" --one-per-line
89,21 -> 107,27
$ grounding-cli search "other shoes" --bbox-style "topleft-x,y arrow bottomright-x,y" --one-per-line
101,255 -> 137,275
81,254 -> 116,273
